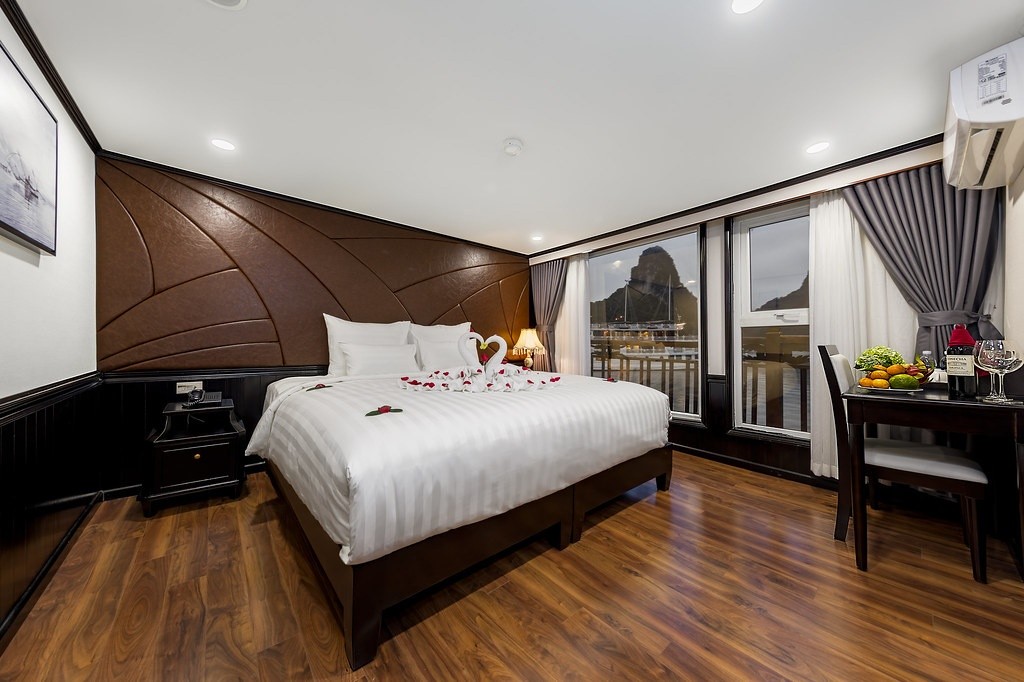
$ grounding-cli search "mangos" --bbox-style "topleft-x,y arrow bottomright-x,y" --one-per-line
859,364 -> 905,388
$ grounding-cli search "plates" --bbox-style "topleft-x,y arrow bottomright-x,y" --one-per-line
857,385 -> 924,395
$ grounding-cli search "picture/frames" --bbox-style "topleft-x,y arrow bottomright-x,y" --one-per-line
0,40 -> 58,258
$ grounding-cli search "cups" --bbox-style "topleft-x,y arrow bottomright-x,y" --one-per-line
929,368 -> 948,383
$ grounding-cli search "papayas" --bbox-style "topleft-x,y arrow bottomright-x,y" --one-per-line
889,373 -> 919,390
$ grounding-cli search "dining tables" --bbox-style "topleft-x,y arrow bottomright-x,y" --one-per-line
840,382 -> 1023,578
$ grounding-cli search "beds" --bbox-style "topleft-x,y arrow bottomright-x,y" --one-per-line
242,364 -> 675,674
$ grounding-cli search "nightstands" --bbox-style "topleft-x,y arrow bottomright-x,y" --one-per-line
135,400 -> 248,517
507,360 -> 532,370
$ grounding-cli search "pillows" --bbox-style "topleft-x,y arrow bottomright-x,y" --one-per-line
415,338 -> 482,371
408,321 -> 472,369
322,313 -> 411,377
337,341 -> 420,379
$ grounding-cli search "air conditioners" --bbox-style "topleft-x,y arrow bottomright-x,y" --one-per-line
940,33 -> 1024,191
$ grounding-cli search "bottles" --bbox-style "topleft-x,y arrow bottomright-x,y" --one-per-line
918,350 -> 937,367
940,350 -> 947,370
946,324 -> 976,402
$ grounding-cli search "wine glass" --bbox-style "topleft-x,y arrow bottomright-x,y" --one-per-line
972,339 -> 1024,405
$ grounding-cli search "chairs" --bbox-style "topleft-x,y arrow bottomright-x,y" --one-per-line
817,342 -> 988,585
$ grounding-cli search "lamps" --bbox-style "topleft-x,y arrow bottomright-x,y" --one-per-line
512,328 -> 546,368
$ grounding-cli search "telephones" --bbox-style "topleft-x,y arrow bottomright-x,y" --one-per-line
188,389 -> 222,408
